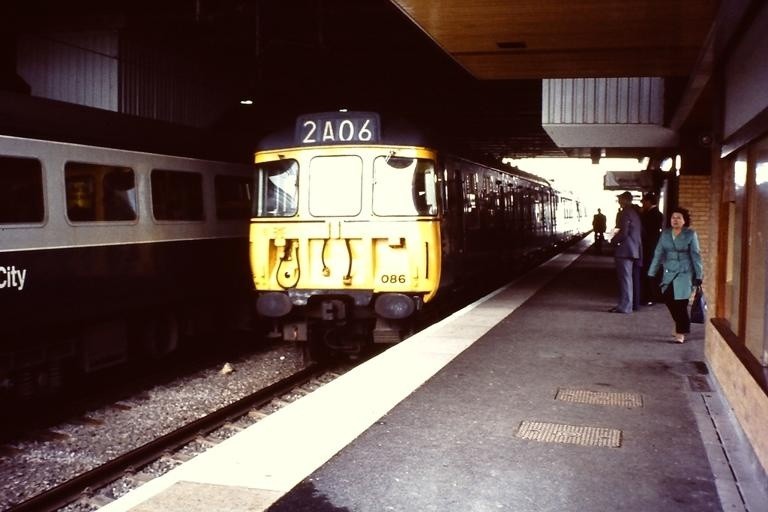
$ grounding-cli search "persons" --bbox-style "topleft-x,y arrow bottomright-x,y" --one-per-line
646,206 -> 708,343
592,207 -> 607,248
608,191 -> 665,313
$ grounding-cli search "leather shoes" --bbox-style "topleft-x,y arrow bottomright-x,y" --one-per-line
608,301 -> 656,313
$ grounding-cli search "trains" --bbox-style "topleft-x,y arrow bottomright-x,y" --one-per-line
244,107 -> 599,367
1,73 -> 257,439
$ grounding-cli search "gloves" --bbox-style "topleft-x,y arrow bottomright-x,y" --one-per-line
694,279 -> 702,286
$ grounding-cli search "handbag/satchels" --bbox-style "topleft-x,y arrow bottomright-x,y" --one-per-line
690,285 -> 708,324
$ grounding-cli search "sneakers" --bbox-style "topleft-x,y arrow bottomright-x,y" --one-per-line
672,327 -> 687,343
